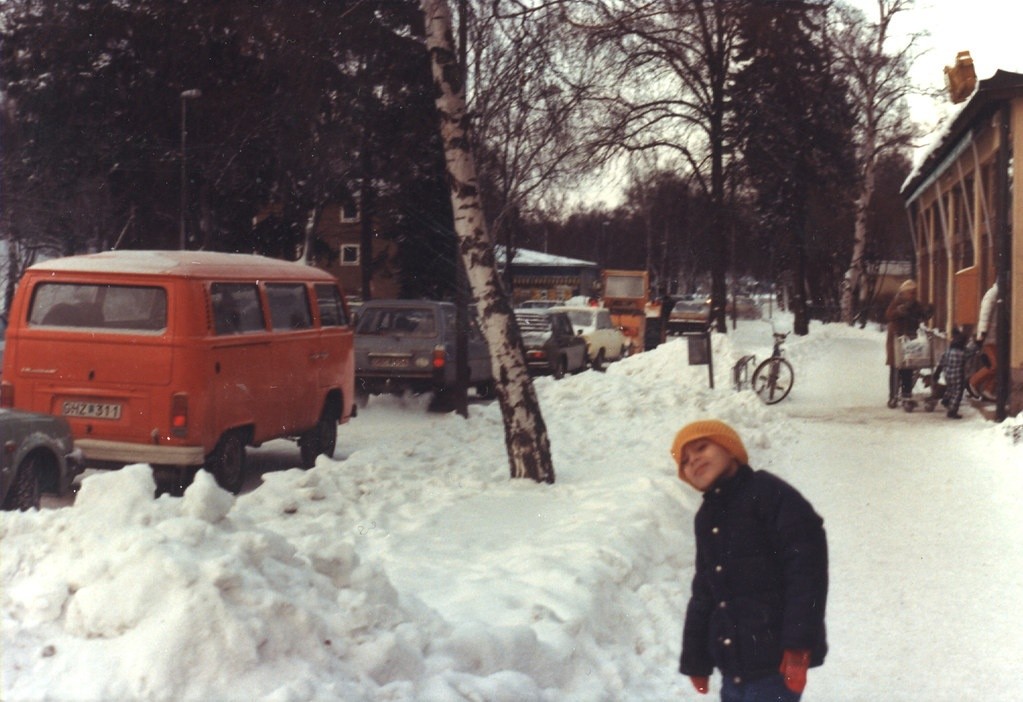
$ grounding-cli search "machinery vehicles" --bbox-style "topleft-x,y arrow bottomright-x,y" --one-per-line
590,268 -> 667,356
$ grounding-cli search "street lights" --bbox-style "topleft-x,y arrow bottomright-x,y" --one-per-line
180,88 -> 205,249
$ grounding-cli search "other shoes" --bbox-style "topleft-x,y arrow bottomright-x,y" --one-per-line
947,411 -> 962,418
940,398 -> 950,409
888,399 -> 897,408
963,375 -> 979,399
981,391 -> 997,402
903,400 -> 918,412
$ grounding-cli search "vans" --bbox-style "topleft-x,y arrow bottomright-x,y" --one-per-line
0,248 -> 358,496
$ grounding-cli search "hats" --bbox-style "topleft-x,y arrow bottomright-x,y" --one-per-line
671,420 -> 748,491
900,279 -> 918,292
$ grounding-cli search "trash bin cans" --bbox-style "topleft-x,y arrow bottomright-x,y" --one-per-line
682,327 -> 713,365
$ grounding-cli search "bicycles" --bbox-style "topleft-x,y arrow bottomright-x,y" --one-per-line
730,317 -> 794,404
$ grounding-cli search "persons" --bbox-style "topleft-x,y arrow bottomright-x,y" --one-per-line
934,334 -> 979,420
670,420 -> 828,702
884,279 -> 933,406
966,275 -> 1001,402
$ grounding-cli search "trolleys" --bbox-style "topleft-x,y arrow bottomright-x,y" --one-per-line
887,328 -> 940,412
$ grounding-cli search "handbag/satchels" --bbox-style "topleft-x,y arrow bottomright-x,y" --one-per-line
899,322 -> 931,367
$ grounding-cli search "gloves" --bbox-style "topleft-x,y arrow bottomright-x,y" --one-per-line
689,675 -> 708,694
780,650 -> 809,692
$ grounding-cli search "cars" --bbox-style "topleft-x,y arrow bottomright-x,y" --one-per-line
0,408 -> 86,513
647,292 -> 762,334
355,293 -> 630,414
